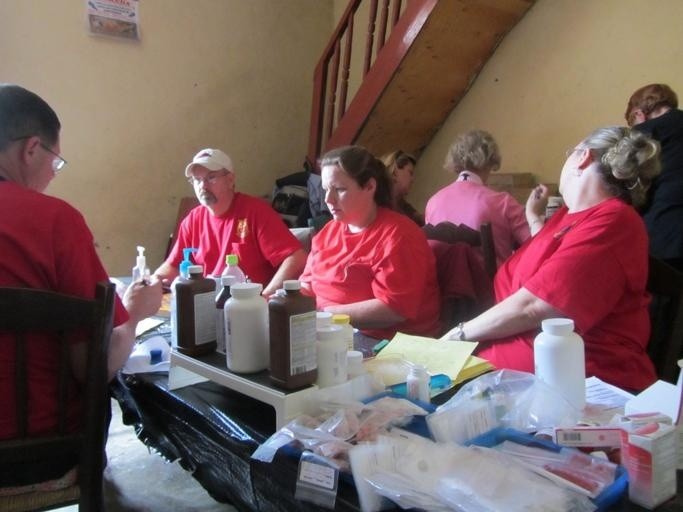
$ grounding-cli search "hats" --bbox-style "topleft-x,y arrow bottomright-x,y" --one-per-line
182,148 -> 235,178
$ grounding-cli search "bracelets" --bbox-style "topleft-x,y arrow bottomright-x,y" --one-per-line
528,218 -> 542,230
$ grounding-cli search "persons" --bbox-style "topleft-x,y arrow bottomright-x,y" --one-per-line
155,148 -> 307,302
437,125 -> 658,392
625,82 -> 683,385
425,128 -> 531,306
0,82 -> 161,487
381,150 -> 425,228
298,145 -> 441,342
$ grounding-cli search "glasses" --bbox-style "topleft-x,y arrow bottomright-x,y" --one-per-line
12,134 -> 69,170
185,175 -> 230,183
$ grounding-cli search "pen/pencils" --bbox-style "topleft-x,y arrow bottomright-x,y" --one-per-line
536,189 -> 540,197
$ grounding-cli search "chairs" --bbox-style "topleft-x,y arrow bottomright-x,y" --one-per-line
421,220 -> 499,339
0,282 -> 117,512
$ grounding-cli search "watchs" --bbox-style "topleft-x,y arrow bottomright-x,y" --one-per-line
457,321 -> 466,342
